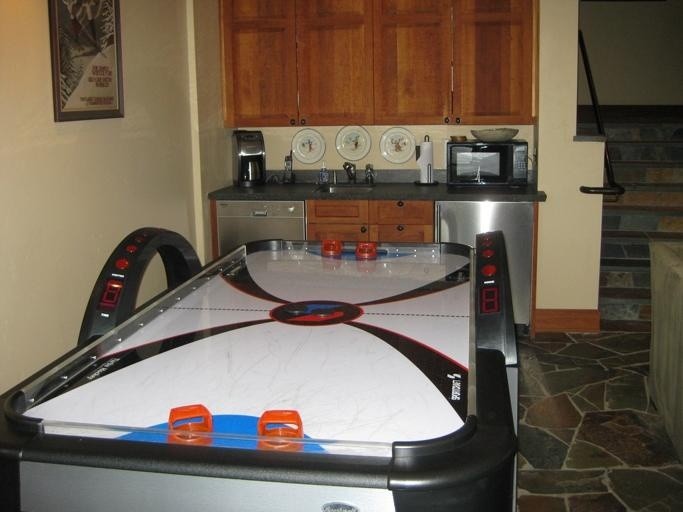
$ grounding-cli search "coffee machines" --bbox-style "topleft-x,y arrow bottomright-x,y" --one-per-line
230,129 -> 268,189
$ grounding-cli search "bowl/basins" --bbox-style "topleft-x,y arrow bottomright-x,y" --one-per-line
449,135 -> 466,141
470,127 -> 519,142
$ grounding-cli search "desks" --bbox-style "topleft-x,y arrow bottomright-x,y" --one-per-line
0,224 -> 521,509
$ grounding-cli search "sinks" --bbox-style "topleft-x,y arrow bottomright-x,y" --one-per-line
310,182 -> 374,195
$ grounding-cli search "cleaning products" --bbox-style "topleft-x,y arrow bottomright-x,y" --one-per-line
318,161 -> 330,184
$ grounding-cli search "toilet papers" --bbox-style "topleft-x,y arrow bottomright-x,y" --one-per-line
417,142 -> 433,184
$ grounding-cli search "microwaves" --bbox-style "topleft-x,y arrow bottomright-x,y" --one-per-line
445,138 -> 528,190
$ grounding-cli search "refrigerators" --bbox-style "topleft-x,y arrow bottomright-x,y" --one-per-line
431,200 -> 532,328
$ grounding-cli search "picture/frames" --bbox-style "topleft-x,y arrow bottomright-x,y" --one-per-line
47,1 -> 124,123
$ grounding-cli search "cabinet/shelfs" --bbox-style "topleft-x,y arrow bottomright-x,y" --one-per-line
373,0 -> 538,126
219,0 -> 373,128
304,201 -> 435,244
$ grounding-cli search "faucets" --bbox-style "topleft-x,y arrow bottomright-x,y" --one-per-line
342,161 -> 357,183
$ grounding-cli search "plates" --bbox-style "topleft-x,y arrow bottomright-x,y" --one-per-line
334,123 -> 371,161
290,128 -> 326,164
378,126 -> 417,164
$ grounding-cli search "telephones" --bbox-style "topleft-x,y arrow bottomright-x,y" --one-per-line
281,149 -> 295,183
